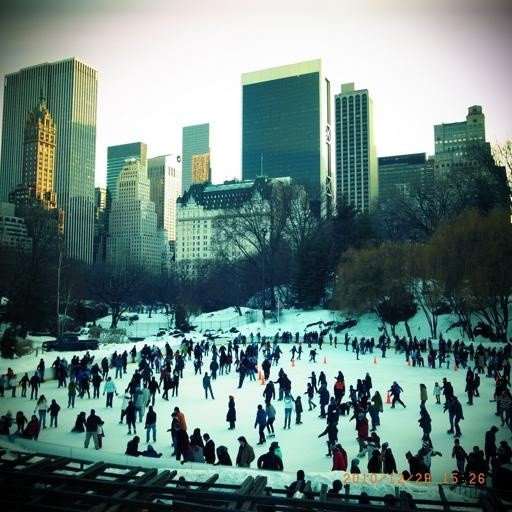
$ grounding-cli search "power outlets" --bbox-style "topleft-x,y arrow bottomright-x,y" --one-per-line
42,334 -> 98,352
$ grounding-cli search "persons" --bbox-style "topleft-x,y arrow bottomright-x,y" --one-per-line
344,332 -> 391,360
176,476 -> 189,488
255,332 -> 337,381
452,426 -> 510,485
194,332 -> 259,389
401,377 -> 464,482
327,480 -> 343,495
394,334 -> 474,371
465,367 -> 480,405
202,373 -> 215,399
286,470 -> 315,501
304,371 -> 406,474
255,368 -> 304,471
384,494 -> 396,505
0,338 -> 193,458
407,494 -> 417,508
473,337 -> 510,430
168,407 -> 256,474
359,492 -> 369,504
226,395 -> 237,430
